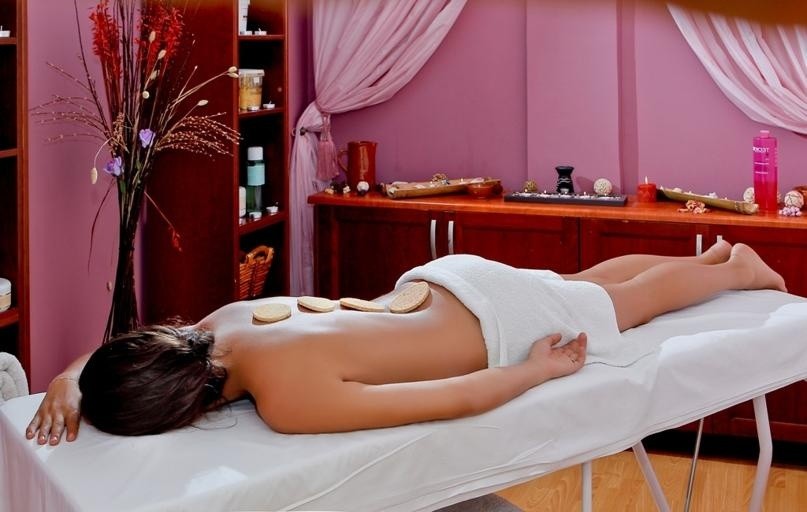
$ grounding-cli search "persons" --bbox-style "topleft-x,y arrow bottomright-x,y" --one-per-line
21,237 -> 793,447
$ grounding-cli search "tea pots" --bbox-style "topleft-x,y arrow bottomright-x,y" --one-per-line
338,141 -> 378,192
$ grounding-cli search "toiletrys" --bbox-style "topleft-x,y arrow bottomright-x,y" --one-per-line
751,130 -> 779,216
245,146 -> 267,213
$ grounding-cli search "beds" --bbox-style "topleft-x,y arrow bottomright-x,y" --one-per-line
0,290 -> 807,512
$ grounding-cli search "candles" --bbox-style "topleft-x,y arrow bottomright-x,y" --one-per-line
637,176 -> 657,204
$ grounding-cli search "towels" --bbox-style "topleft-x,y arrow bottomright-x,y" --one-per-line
0,353 -> 29,406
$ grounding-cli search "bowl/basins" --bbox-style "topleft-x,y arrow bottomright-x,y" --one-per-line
466,183 -> 494,200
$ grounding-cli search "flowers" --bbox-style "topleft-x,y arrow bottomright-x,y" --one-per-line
26,0 -> 239,345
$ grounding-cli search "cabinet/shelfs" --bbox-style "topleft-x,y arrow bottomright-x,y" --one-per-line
579,191 -> 806,447
141,0 -> 292,327
0,0 -> 32,396
304,191 -> 580,302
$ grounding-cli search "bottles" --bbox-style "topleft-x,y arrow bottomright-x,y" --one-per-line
556,166 -> 574,194
247,146 -> 265,212
752,129 -> 777,211
